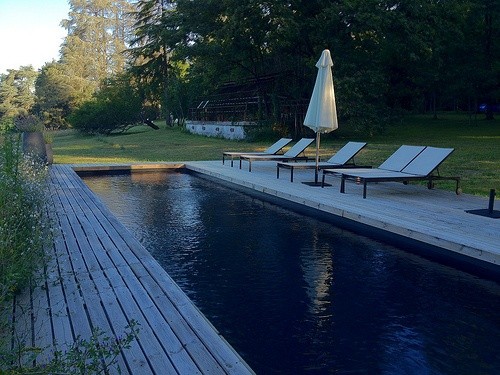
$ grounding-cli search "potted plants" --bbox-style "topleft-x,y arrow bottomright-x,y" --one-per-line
43,131 -> 53,166
16,115 -> 48,167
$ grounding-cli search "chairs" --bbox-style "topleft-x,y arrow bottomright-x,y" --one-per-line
341,146 -> 460,199
239,137 -> 316,172
322,144 -> 428,193
277,141 -> 368,182
223,138 -> 293,167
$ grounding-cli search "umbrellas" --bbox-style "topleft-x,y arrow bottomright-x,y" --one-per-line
303,48 -> 340,184
